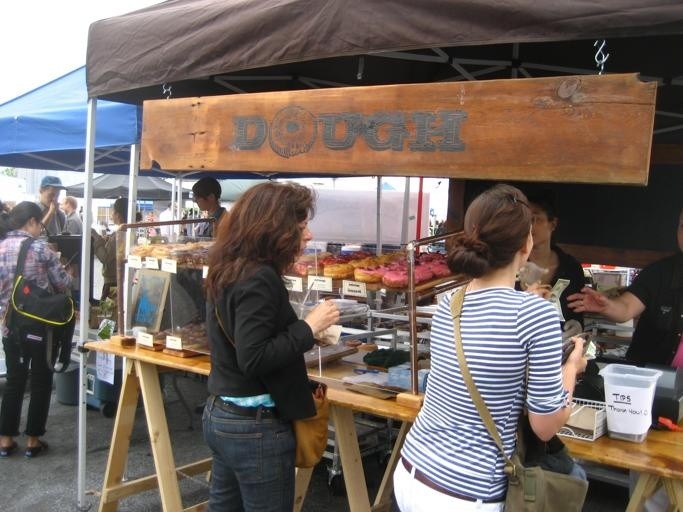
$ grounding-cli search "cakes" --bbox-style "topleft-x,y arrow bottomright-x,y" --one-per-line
291,250 -> 452,288
132,240 -> 217,265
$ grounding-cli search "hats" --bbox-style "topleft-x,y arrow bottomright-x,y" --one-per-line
41,176 -> 67,190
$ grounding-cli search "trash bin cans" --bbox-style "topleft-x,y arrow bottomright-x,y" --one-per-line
55,358 -> 80,406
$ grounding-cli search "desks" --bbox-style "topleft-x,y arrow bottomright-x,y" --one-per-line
82,317 -> 683,512
123,242 -> 464,318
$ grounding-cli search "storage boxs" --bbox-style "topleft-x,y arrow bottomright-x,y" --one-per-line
298,241 -> 361,257
84,364 -> 164,413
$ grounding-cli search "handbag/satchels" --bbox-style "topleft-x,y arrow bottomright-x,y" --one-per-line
4,275 -> 75,351
505,414 -> 589,511
292,378 -> 329,467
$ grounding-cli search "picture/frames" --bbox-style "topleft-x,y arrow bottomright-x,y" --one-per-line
128,268 -> 171,336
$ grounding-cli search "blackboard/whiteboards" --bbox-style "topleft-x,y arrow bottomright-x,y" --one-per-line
131,268 -> 171,334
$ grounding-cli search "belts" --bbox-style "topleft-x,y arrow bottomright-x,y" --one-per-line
214,399 -> 276,418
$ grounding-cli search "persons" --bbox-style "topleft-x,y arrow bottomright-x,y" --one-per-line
202,181 -> 340,511
392,183 -> 588,512
514,202 -> 584,344
160,202 -> 181,244
0,202 -> 75,458
91,198 -> 142,303
0,176 -> 84,250
567,208 -> 682,512
179,177 -> 228,238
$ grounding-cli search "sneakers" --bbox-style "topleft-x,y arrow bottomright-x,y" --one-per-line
0,441 -> 17,458
25,440 -> 48,458
